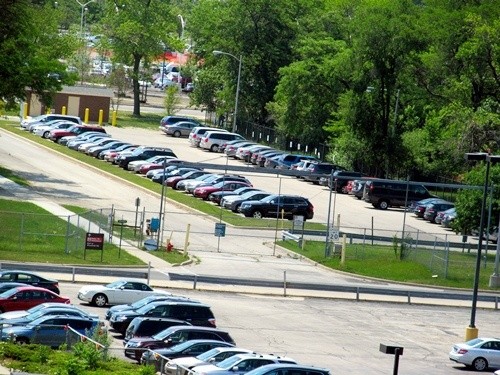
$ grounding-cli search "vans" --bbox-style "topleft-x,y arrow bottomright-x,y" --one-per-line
122,318 -> 194,348
363,180 -> 440,210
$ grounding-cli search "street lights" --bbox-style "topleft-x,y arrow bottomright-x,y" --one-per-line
379,343 -> 403,375
211,50 -> 243,132
462,152 -> 500,341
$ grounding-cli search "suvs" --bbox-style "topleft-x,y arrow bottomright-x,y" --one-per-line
108,300 -> 217,337
104,295 -> 201,321
239,194 -> 314,222
124,325 -> 236,363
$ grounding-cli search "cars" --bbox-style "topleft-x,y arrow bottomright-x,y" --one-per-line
0,286 -> 71,312
0,302 -> 100,334
164,347 -> 255,375
239,363 -> 331,375
46,29 -> 196,93
0,282 -> 35,295
0,314 -> 108,350
140,339 -> 237,372
0,269 -> 60,296
77,280 -> 172,307
19,113 -> 382,214
411,198 -> 458,229
449,337 -> 500,371
187,352 -> 298,375
